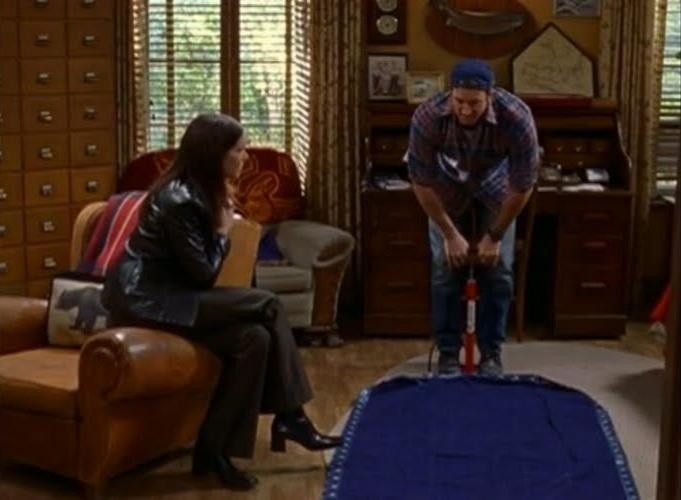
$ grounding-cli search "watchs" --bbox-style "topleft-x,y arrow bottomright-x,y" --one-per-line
486,228 -> 503,242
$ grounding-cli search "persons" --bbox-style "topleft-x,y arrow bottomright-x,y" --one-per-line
407,58 -> 541,377
101,115 -> 345,491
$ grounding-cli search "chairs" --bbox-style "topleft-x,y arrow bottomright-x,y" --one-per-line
122,146 -> 356,351
0,198 -> 265,499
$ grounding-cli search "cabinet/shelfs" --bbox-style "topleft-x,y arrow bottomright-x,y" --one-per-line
0,0 -> 119,299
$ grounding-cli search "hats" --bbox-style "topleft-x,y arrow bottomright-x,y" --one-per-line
451,59 -> 492,92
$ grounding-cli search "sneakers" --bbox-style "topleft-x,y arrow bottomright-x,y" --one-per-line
437,350 -> 459,379
479,351 -> 502,379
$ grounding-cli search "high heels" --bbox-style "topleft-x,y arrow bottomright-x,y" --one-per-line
192,449 -> 257,491
270,410 -> 342,453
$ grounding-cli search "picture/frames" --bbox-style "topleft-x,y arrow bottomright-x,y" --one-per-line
404,69 -> 446,104
367,52 -> 409,101
364,0 -> 409,47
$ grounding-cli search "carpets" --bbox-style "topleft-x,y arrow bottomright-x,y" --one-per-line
320,341 -> 665,500
320,368 -> 639,499
